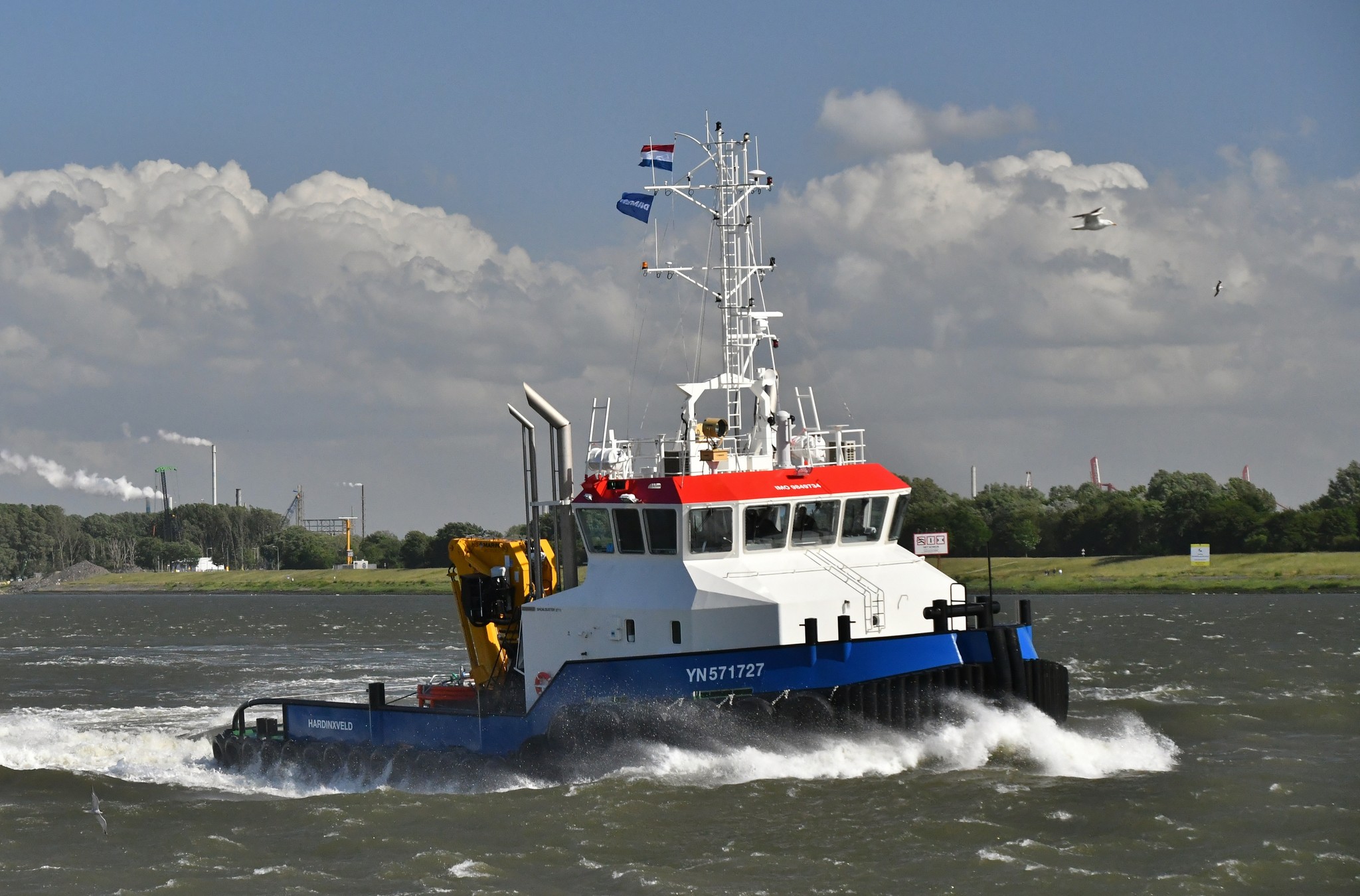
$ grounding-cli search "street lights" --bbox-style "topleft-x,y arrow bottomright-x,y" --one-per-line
272,546 -> 279,570
207,547 -> 213,558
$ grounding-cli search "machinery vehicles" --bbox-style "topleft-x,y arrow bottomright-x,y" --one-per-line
154,466 -> 178,572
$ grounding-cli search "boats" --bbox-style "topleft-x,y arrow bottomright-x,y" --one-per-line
208,0 -> 1072,791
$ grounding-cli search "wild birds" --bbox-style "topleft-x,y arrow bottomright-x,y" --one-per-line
1212,278 -> 1224,297
81,783 -> 110,839
1067,205 -> 1118,232
